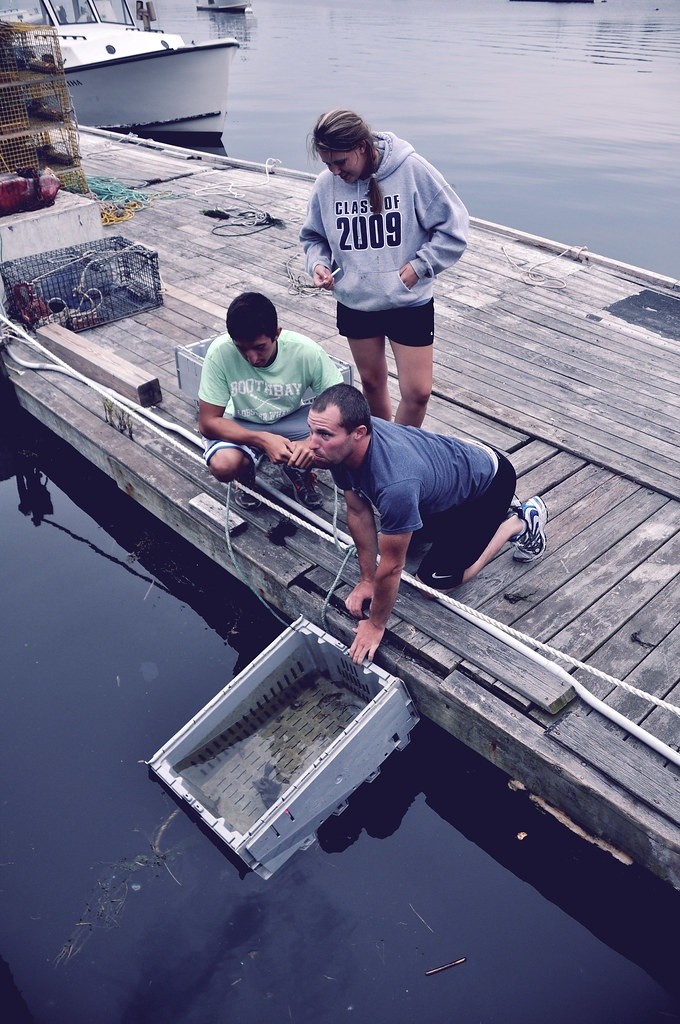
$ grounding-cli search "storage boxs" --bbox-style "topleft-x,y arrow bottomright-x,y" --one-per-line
173,332 -> 353,413
147,614 -> 420,879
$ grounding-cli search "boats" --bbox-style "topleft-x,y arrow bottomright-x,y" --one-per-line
0,0 -> 242,152
197,0 -> 249,15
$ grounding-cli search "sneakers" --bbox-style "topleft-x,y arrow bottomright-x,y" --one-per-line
513,496 -> 548,563
507,494 -> 525,542
280,461 -> 324,509
234,464 -> 262,509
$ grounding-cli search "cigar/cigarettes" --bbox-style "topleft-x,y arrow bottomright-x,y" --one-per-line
324,267 -> 341,281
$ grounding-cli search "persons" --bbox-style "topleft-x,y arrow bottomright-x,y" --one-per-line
197,292 -> 344,508
298,108 -> 470,426
306,383 -> 547,665
